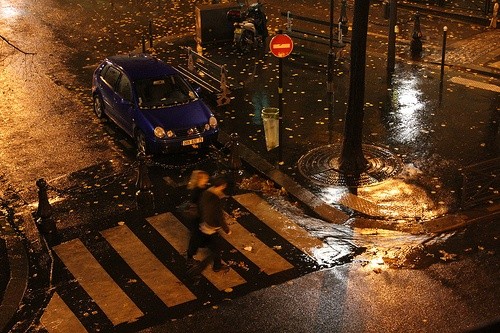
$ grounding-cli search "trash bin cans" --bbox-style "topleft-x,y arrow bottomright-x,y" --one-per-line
261,108 -> 280,152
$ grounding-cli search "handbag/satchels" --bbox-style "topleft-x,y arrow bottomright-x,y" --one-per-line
176,194 -> 200,219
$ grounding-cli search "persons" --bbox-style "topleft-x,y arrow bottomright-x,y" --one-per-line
198,176 -> 232,272
180,170 -> 209,266
489,0 -> 499,27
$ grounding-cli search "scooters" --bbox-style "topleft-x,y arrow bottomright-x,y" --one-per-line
226,2 -> 268,52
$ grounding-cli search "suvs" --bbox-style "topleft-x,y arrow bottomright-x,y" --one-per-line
92,56 -> 219,159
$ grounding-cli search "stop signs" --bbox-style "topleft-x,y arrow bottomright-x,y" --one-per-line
270,34 -> 293,58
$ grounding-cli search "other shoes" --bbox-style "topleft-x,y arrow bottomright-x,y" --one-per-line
186,259 -> 200,266
212,264 -> 230,272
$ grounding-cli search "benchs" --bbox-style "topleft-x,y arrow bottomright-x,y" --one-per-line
171,46 -> 245,108
276,11 -> 353,61
